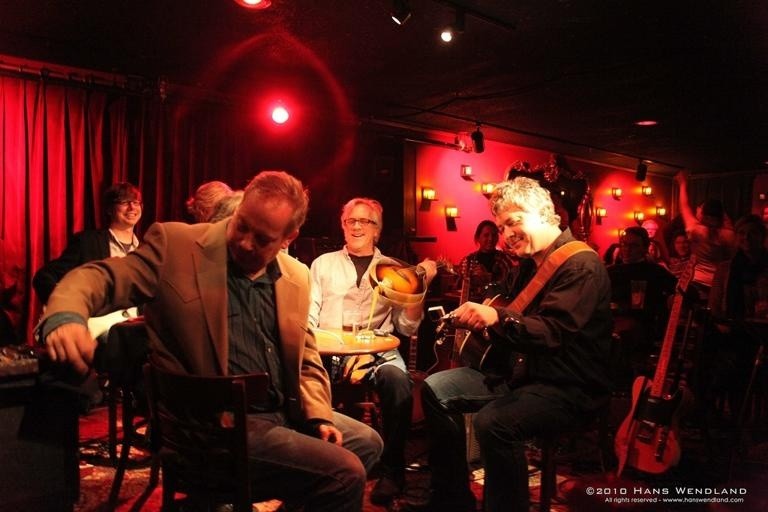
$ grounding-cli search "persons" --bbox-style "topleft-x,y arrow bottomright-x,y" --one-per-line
30,168 -> 768,512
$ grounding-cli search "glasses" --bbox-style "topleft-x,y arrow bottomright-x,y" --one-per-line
119,199 -> 141,206
344,217 -> 375,227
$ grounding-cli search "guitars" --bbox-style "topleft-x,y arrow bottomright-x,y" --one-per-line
427,254 -> 513,375
616,254 -> 698,475
400,329 -> 426,428
